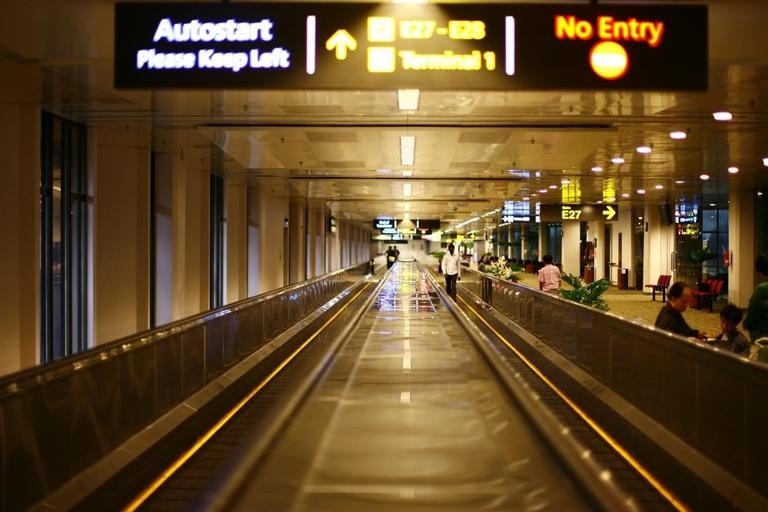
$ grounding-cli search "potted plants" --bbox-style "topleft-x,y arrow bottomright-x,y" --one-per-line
508,274 -> 524,284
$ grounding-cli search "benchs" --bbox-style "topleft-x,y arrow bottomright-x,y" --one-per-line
643,274 -> 722,314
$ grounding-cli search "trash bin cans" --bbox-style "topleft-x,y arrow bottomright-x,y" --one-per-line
584,266 -> 594,283
618,268 -> 628,290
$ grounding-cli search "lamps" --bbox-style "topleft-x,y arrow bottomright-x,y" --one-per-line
396,217 -> 416,235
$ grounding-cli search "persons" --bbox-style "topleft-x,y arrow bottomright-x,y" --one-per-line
385,245 -> 398,270
478,255 -> 489,271
440,242 -> 461,302
538,255 -> 562,296
655,281 -> 713,339
393,245 -> 399,260
714,304 -> 752,353
742,255 -> 768,341
